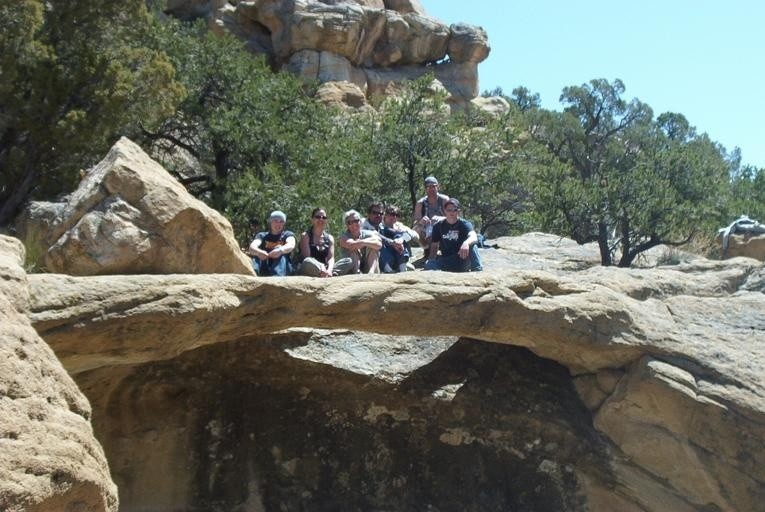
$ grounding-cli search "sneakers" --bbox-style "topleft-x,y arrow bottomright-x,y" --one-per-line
421,248 -> 430,263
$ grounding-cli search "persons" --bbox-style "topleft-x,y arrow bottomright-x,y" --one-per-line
424,198 -> 483,272
246,210 -> 297,277
292,207 -> 353,278
412,177 -> 450,259
381,205 -> 420,270
337,209 -> 383,274
361,203 -> 409,273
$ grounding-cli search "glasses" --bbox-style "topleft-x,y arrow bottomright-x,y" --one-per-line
371,210 -> 384,216
445,206 -> 459,212
347,219 -> 360,225
425,184 -> 438,190
312,215 -> 327,219
387,212 -> 400,218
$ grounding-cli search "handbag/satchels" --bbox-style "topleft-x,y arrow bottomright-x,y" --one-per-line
475,233 -> 490,248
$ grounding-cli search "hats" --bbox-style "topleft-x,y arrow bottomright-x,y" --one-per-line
270,210 -> 287,224
425,177 -> 438,186
